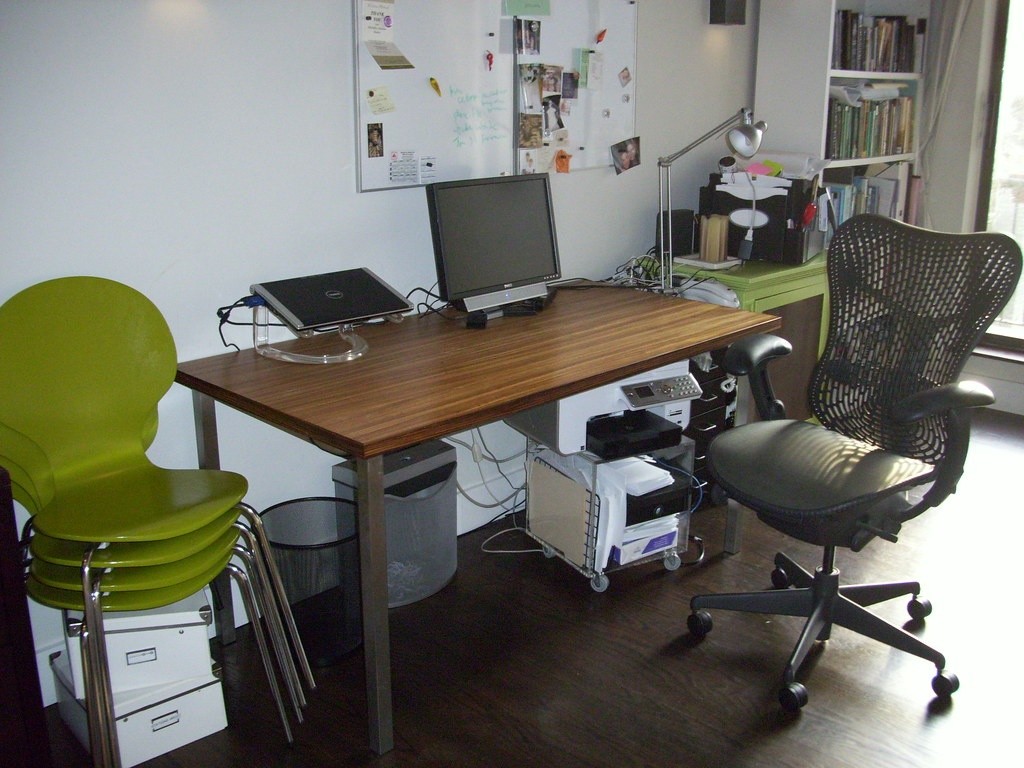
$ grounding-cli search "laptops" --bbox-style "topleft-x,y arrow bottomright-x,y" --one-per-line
250,267 -> 414,333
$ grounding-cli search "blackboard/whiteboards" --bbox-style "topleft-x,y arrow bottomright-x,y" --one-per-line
355,0 -> 640,196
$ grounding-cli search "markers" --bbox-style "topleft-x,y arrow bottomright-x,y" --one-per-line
787,218 -> 795,229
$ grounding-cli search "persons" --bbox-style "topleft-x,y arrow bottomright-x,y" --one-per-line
520,21 -> 561,174
620,68 -> 630,85
612,139 -> 639,172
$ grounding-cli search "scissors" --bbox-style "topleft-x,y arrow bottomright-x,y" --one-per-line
802,201 -> 818,227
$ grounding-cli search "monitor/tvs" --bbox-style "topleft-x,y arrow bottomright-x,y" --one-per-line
425,173 -> 562,301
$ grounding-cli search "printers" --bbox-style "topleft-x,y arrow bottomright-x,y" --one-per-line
500,358 -> 704,459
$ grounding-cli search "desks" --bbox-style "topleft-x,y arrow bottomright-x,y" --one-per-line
636,248 -> 831,426
177,279 -> 784,756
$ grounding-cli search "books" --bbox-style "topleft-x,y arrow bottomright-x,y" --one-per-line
825,11 -> 912,247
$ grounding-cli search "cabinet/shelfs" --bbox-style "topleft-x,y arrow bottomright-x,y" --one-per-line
688,344 -> 738,511
752,0 -> 928,250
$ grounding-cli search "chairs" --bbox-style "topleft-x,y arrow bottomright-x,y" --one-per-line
0,276 -> 316,768
685,213 -> 1023,712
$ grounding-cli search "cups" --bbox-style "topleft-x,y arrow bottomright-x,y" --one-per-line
698,214 -> 729,264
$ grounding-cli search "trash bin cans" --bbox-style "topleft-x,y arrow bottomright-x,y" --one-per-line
331,440 -> 459,615
249,498 -> 363,667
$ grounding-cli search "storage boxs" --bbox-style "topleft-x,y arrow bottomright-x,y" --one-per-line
61,589 -> 213,699
49,649 -> 229,768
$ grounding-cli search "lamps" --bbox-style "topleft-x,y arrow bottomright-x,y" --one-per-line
655,108 -> 767,294
